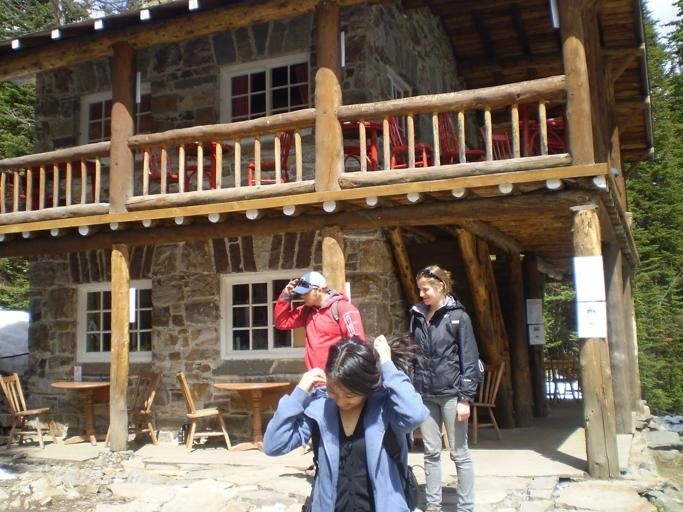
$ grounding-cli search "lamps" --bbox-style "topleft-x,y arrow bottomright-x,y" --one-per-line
139,144 -> 179,194
549,348 -> 583,408
105,370 -> 160,447
0,372 -> 57,449
247,129 -> 293,186
387,109 -> 513,170
176,370 -> 232,453
468,360 -> 505,444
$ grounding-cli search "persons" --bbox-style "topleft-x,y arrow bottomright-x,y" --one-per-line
272,270 -> 366,371
261,329 -> 431,511
404,262 -> 482,511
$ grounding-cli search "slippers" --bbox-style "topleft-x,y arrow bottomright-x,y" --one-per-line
383,424 -> 418,512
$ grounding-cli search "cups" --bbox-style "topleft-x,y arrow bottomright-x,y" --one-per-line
419,269 -> 443,282
297,277 -> 319,290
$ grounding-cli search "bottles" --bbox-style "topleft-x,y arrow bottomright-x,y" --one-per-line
292,272 -> 327,295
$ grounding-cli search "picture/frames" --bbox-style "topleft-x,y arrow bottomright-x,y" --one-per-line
213,382 -> 292,452
22,160 -> 105,209
340,119 -> 384,171
517,117 -> 566,157
50,382 -> 111,446
175,141 -> 233,192
